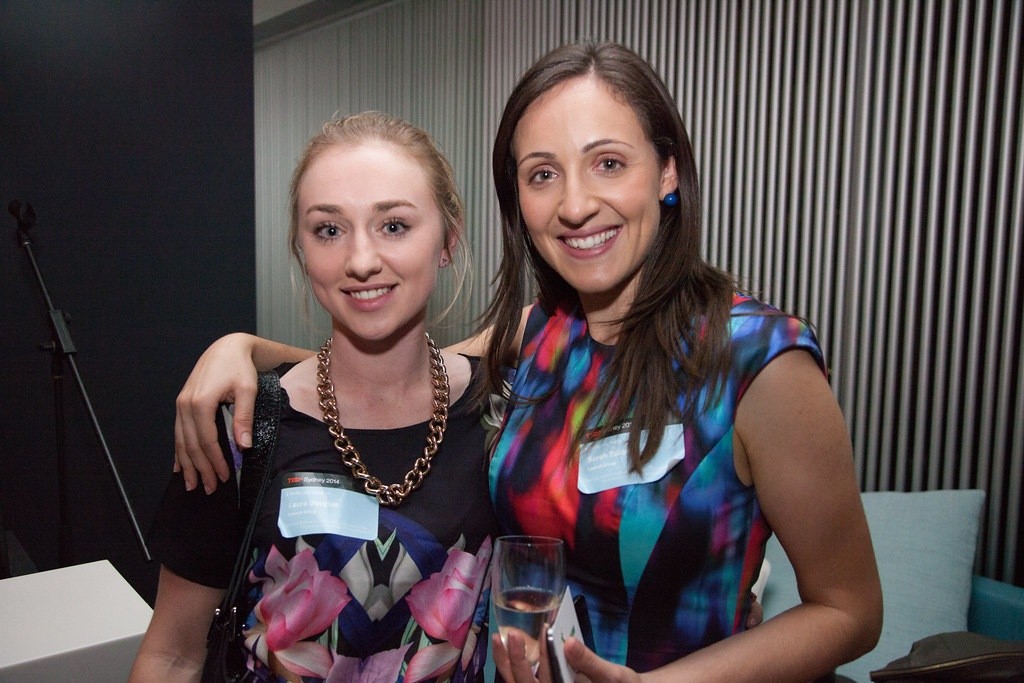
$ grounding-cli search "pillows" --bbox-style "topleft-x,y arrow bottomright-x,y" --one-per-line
752,485 -> 986,683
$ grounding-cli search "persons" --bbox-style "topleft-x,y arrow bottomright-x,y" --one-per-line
125,112 -> 509,683
173,42 -> 884,683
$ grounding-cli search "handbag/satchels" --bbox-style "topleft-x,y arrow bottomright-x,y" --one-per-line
199,373 -> 284,683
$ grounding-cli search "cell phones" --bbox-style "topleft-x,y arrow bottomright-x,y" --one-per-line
546,594 -> 597,683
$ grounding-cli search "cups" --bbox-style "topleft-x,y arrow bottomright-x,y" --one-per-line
492,535 -> 567,683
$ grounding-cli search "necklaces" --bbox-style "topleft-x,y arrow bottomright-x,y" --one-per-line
313,333 -> 452,505
